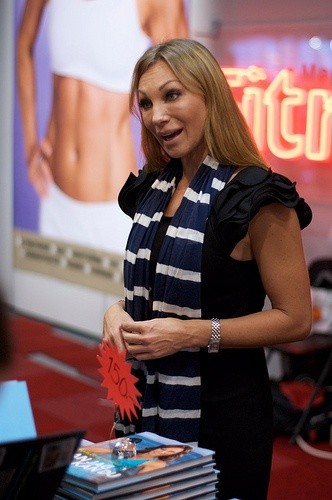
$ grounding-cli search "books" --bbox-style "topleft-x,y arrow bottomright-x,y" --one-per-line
53,431 -> 220,500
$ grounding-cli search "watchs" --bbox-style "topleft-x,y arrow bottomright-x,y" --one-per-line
208,318 -> 221,353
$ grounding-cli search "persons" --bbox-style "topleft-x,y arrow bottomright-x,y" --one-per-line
14,0 -> 191,256
97,38 -> 313,500
78,445 -> 192,475
